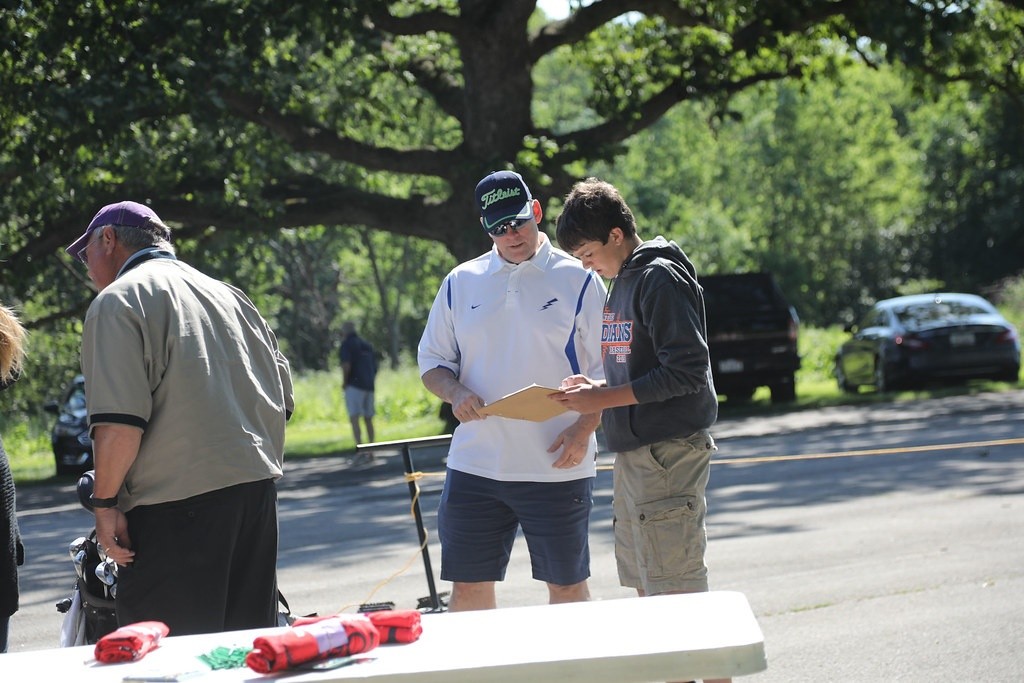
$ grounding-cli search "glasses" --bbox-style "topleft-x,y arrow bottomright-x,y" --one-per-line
488,218 -> 530,237
77,235 -> 104,271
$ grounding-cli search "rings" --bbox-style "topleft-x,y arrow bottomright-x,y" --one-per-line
573,461 -> 578,465
106,547 -> 110,552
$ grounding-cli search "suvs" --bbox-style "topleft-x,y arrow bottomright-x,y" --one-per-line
697,271 -> 802,405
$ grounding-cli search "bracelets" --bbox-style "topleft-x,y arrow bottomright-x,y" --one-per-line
90,493 -> 118,509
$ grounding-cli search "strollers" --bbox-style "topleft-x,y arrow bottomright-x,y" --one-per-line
55,469 -> 118,647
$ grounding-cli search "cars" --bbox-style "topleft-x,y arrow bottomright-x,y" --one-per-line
42,374 -> 95,475
833,291 -> 1021,396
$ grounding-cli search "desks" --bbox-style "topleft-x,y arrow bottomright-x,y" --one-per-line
0,590 -> 769,683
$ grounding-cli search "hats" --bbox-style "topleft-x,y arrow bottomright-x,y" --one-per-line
65,201 -> 170,265
475,170 -> 533,231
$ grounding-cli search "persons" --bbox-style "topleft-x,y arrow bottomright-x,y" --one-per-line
417,172 -> 609,611
1,298 -> 32,653
548,180 -> 719,683
63,201 -> 292,645
338,322 -> 381,444
